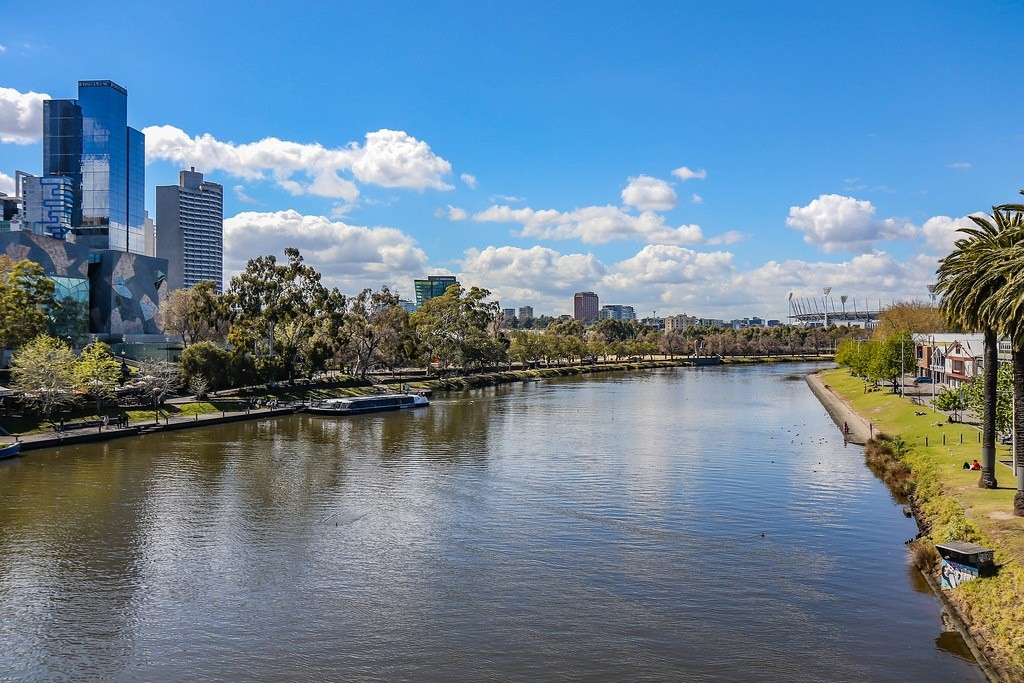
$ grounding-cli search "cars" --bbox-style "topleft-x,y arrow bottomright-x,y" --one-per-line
913,376 -> 932,383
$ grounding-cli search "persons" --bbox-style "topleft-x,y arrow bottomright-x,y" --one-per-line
915,411 -> 980,470
251,396 -> 279,409
864,384 -> 880,394
100,412 -> 128,430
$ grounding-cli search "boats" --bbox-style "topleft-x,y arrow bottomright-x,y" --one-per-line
298,395 -> 430,416
